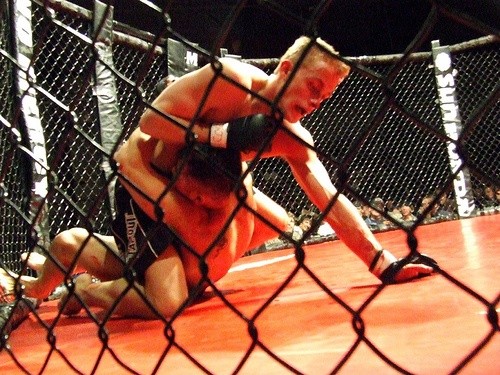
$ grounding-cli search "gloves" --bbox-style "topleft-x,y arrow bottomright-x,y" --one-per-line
208,112 -> 277,154
154,73 -> 177,95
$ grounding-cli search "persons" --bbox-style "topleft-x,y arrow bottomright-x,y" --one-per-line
1,35 -> 500,345
